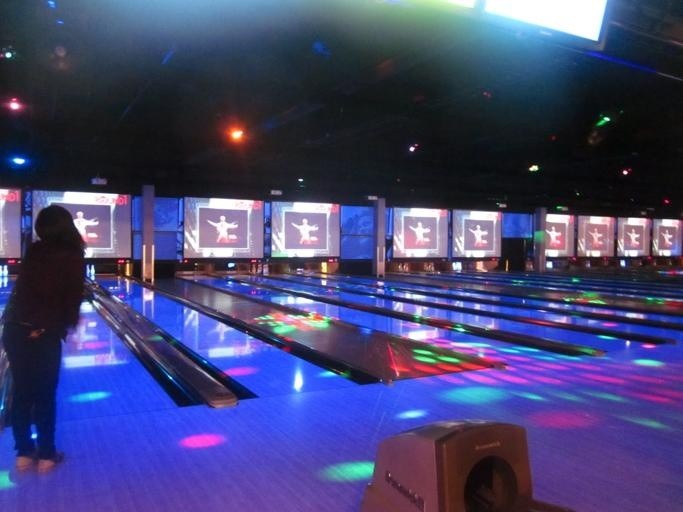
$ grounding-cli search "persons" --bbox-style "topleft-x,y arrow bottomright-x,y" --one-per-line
0,205 -> 88,474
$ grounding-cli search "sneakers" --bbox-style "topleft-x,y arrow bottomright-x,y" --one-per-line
16,451 -> 64,472
